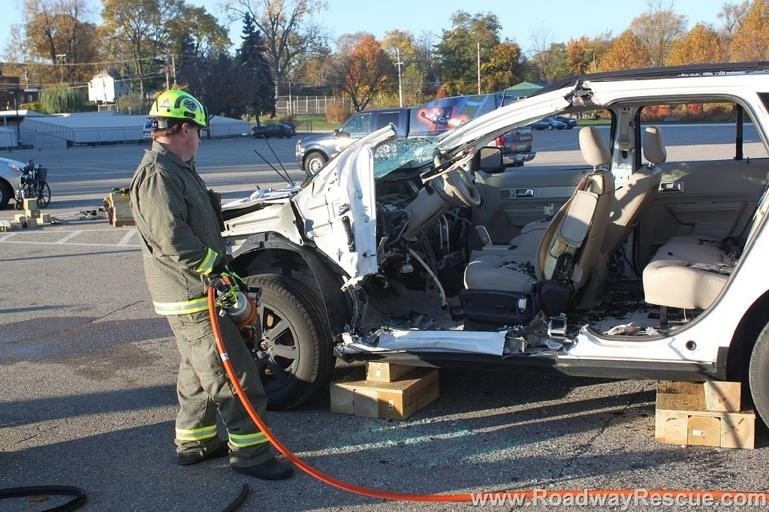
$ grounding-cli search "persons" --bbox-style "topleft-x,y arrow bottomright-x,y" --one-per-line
129,88 -> 293,480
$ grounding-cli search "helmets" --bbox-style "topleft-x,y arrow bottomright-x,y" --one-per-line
149,89 -> 207,127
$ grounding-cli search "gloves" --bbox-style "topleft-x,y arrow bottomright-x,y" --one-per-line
219,271 -> 250,309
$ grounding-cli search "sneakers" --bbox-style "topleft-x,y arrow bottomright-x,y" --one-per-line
176,439 -> 229,465
230,457 -> 293,480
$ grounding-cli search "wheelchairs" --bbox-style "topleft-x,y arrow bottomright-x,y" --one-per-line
15,161 -> 52,210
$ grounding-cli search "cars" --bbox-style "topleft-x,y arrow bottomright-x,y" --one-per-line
0,156 -> 27,210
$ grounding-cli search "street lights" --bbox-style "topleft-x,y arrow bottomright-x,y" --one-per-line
21,46 -> 29,88
56,54 -> 66,82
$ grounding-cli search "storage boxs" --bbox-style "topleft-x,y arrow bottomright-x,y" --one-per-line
652,379 -> 757,451
107,191 -> 134,227
327,357 -> 440,421
1,199 -> 51,231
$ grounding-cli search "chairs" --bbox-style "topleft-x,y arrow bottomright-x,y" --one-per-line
466,119 -> 736,332
